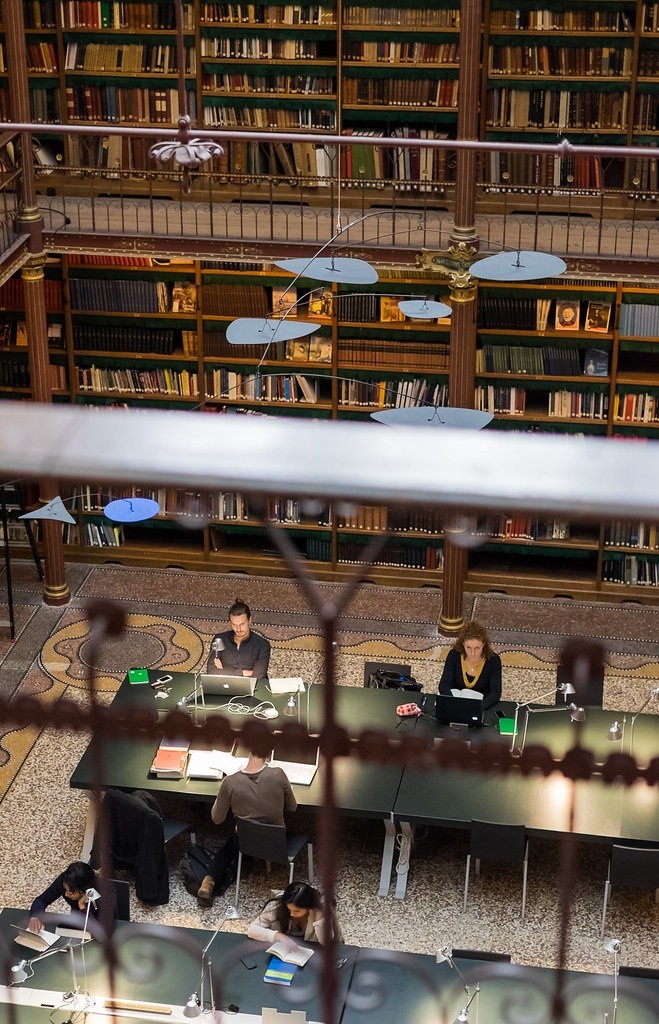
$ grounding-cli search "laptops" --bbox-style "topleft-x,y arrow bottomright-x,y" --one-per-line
435,694 -> 486,724
269,731 -> 320,785
201,674 -> 257,697
9,923 -> 60,946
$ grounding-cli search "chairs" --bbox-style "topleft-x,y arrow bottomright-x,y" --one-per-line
556,639 -> 604,709
452,949 -> 511,964
364,662 -> 411,688
619,966 -> 659,979
102,789 -> 197,904
464,818 -> 529,919
235,816 -> 313,907
601,844 -> 659,939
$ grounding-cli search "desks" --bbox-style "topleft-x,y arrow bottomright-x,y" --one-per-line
0,667 -> 659,1024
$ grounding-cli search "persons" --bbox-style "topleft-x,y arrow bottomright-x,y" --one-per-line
197,739 -> 297,906
439,621 -> 502,710
207,599 -> 271,680
248,882 -> 344,945
28,861 -> 119,943
560,306 -> 575,326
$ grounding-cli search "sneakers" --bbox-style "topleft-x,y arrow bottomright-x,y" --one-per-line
198,876 -> 215,906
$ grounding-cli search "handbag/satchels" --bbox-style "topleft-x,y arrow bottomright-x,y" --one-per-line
367,670 -> 423,692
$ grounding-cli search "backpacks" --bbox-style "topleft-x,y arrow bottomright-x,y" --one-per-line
181,842 -> 235,898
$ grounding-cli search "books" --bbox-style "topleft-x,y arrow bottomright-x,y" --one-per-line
263,956 -> 298,986
265,942 -> 314,967
128,669 -> 149,684
499,718 -> 518,735
0,0 -> 659,200
150,736 -> 237,780
0,255 -> 659,586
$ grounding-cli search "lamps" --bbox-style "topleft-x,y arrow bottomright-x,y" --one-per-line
510,682 -> 585,759
8,887 -> 102,1024
183,906 -> 241,1023
194,638 -> 225,727
604,939 -> 622,1024
609,689 -> 659,757
287,643 -> 339,734
435,947 -> 480,1024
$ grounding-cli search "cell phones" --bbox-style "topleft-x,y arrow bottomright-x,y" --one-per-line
494,711 -> 507,719
240,958 -> 257,970
157,675 -> 172,684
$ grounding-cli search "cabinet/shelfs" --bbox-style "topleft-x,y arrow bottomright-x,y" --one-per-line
0,0 -> 658,606
